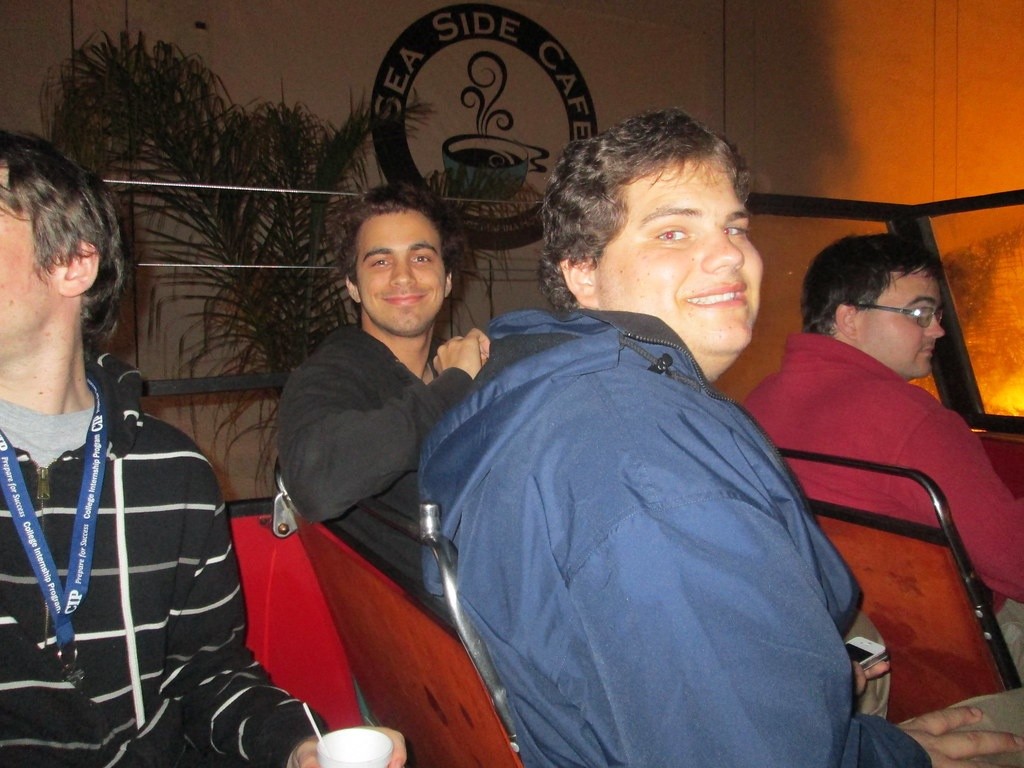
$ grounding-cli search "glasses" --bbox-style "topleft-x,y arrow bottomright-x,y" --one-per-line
850,301 -> 943,328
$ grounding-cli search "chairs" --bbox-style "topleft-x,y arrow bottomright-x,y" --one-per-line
777,447 -> 1022,723
272,459 -> 522,768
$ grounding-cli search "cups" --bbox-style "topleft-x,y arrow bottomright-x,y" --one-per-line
316,728 -> 393,768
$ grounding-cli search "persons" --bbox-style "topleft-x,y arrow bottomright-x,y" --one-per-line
747,235 -> 1024,677
415,102 -> 1024,768
0,128 -> 410,768
280,174 -> 490,575
